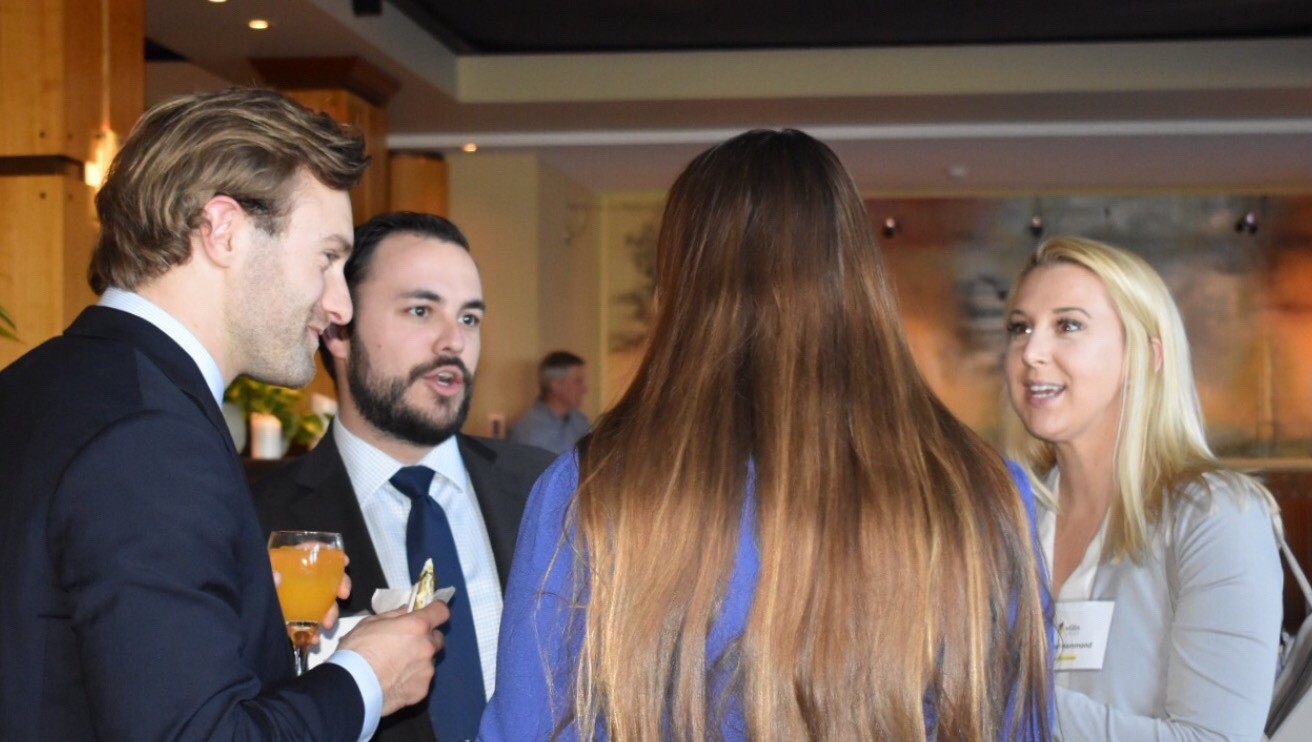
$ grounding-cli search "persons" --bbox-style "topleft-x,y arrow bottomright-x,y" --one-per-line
251,212 -> 558,742
1004,234 -> 1283,742
508,350 -> 593,456
0,85 -> 450,742
473,127 -> 1057,742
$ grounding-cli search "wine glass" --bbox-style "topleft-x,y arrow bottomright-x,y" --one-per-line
268,529 -> 345,676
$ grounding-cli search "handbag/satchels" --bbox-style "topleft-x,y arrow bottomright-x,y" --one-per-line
1165,470 -> 1312,741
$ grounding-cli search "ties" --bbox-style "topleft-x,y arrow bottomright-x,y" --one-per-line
388,465 -> 487,742
